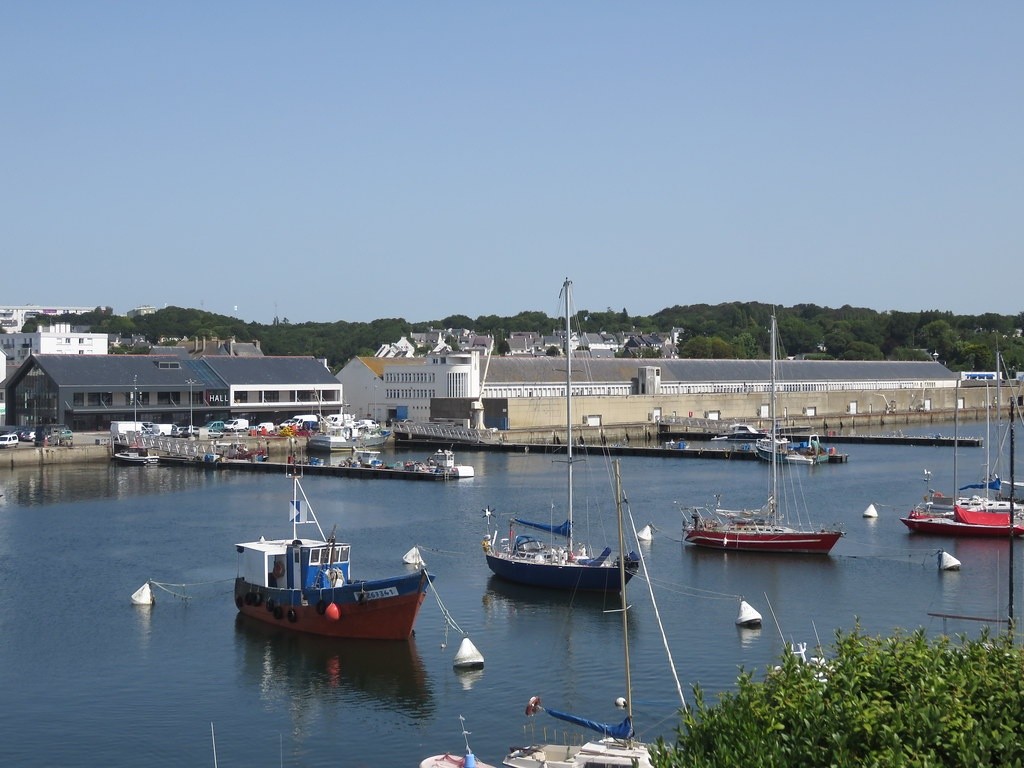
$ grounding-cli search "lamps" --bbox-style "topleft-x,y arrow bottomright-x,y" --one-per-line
929,489 -> 935,499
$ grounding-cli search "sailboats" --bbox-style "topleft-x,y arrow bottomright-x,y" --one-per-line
899,333 -> 1024,537
679,312 -> 848,555
485,273 -> 645,593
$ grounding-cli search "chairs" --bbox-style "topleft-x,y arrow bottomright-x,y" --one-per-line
578,546 -> 612,567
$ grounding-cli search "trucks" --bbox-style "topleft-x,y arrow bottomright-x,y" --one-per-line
110,415 -> 413,439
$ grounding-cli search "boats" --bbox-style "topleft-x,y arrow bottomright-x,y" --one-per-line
754,427 -> 830,466
710,435 -> 728,442
418,463 -> 681,768
114,450 -> 160,462
725,423 -> 766,438
234,444 -> 436,642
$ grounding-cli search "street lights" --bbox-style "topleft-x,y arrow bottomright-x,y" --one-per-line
185,378 -> 198,441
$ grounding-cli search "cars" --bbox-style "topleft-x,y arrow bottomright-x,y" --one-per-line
0,429 -> 74,450
